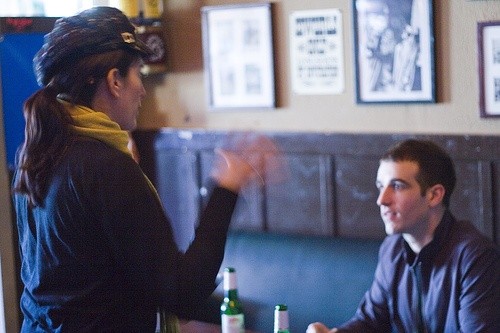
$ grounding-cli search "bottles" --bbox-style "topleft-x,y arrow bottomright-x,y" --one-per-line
221,268 -> 244,333
273,304 -> 290,333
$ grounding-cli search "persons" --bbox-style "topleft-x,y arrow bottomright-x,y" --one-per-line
10,6 -> 285,333
305,139 -> 500,333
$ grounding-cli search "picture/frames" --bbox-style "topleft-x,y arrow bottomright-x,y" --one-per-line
202,3 -> 277,111
477,21 -> 500,117
351,0 -> 437,104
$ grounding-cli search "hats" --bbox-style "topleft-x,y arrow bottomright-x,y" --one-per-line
33,6 -> 156,88
